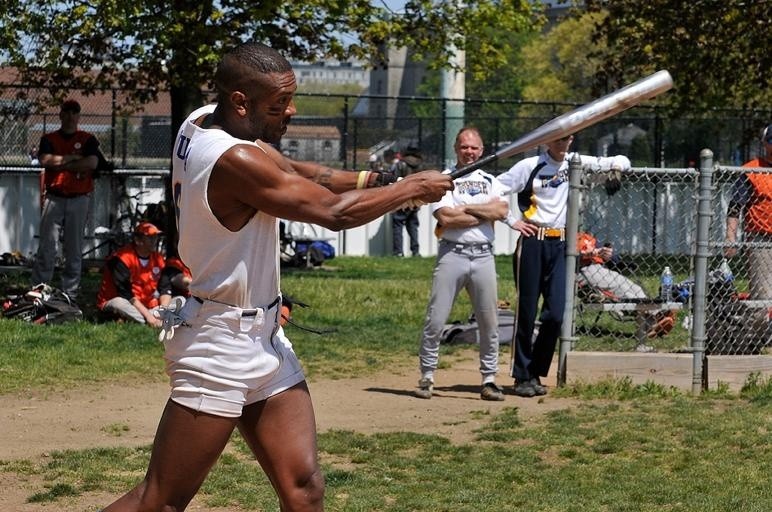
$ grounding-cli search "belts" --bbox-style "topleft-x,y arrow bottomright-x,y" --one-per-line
542,227 -> 563,237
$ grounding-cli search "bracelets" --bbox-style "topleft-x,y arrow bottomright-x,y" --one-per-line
358,170 -> 372,188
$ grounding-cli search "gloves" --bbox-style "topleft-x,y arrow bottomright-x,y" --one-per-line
605,169 -> 621,195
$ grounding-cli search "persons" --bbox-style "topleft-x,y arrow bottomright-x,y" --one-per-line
417,126 -> 509,401
97,42 -> 456,512
577,127 -> 771,346
494,133 -> 632,396
31,102 -> 192,329
368,149 -> 423,257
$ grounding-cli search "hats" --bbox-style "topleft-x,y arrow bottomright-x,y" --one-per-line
136,224 -> 161,235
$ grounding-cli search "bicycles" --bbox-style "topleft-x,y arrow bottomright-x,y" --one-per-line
105,187 -> 173,261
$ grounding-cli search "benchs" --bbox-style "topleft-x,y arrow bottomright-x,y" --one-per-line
576,300 -> 772,351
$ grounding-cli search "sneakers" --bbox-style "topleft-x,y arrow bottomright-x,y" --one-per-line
514,378 -> 545,396
481,382 -> 504,400
416,377 -> 433,399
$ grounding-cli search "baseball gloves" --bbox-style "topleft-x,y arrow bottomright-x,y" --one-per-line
2,282 -> 84,325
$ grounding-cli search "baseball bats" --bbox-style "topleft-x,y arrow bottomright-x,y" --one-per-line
449,70 -> 673,179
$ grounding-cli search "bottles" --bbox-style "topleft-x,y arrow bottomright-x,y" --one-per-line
660,265 -> 674,303
717,257 -> 735,280
27,252 -> 33,266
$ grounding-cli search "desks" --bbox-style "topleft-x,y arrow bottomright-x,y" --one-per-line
289,236 -> 336,270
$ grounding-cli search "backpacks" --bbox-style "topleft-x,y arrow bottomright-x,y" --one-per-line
1,284 -> 84,325
